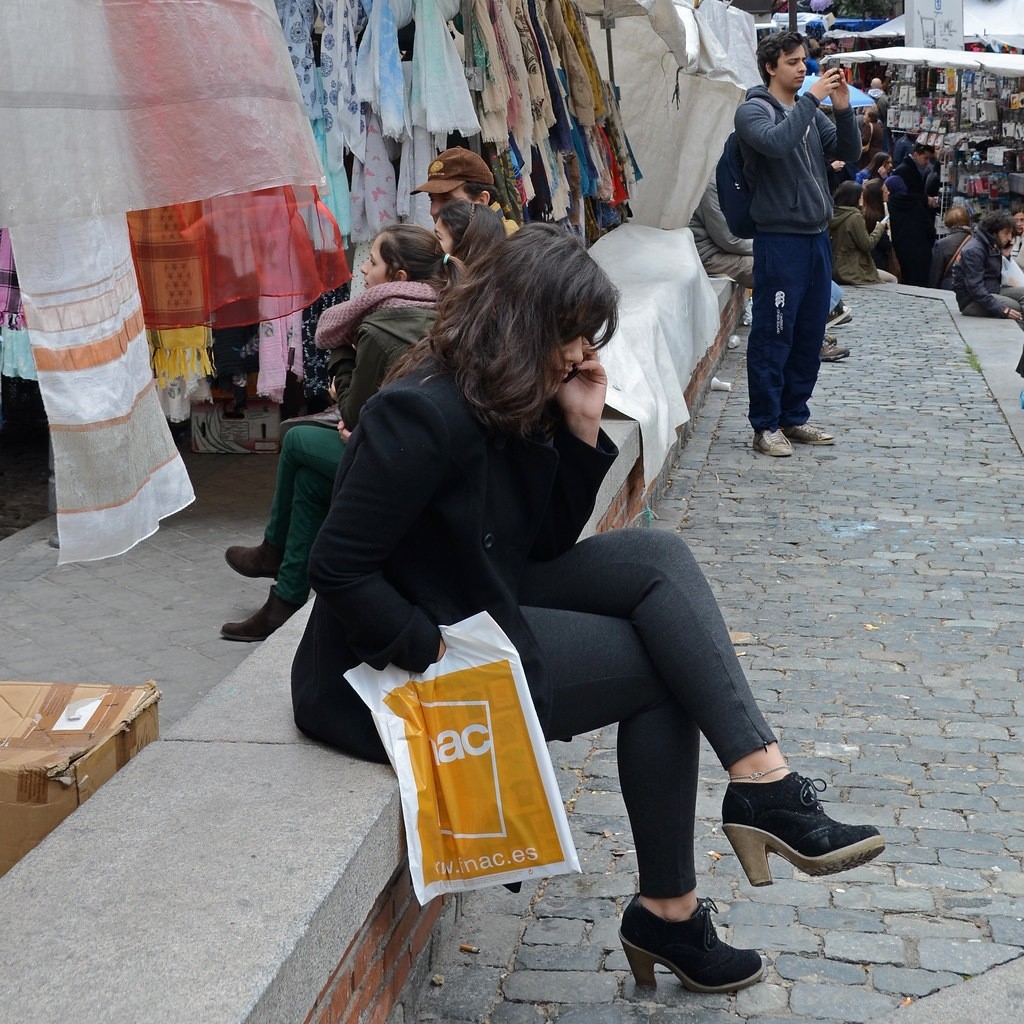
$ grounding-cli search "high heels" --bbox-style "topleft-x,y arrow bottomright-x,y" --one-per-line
618,893 -> 764,993
720,772 -> 885,887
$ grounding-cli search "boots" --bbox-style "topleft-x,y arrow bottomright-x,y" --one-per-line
226,545 -> 283,581
220,586 -> 304,640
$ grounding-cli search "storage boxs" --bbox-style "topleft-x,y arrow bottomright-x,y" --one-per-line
190,395 -> 282,455
0,680 -> 163,877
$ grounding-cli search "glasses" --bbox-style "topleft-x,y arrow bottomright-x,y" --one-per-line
558,317 -> 598,342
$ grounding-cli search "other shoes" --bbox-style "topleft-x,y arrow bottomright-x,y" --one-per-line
826,299 -> 852,330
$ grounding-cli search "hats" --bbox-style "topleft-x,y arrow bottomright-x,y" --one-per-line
885,175 -> 907,196
409,146 -> 495,196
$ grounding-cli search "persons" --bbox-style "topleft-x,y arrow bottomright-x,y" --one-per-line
930,207 -> 970,288
735,31 -> 863,456
954,210 -> 1024,332
857,111 -> 942,288
291,224 -> 886,990
689,177 -> 852,360
1010,205 -> 1024,269
858,78 -> 888,121
221,145 -> 519,642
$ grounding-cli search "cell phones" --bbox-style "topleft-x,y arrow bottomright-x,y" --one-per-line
562,364 -> 584,384
826,58 -> 840,90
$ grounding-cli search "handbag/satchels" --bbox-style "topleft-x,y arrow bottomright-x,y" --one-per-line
1001,255 -> 1024,288
862,142 -> 871,152
343,610 -> 582,906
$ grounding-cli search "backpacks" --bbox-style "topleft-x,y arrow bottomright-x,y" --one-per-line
716,97 -> 775,239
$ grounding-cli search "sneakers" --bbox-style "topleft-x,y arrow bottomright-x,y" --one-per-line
782,422 -> 836,444
823,335 -> 838,346
753,429 -> 794,456
821,345 -> 850,360
